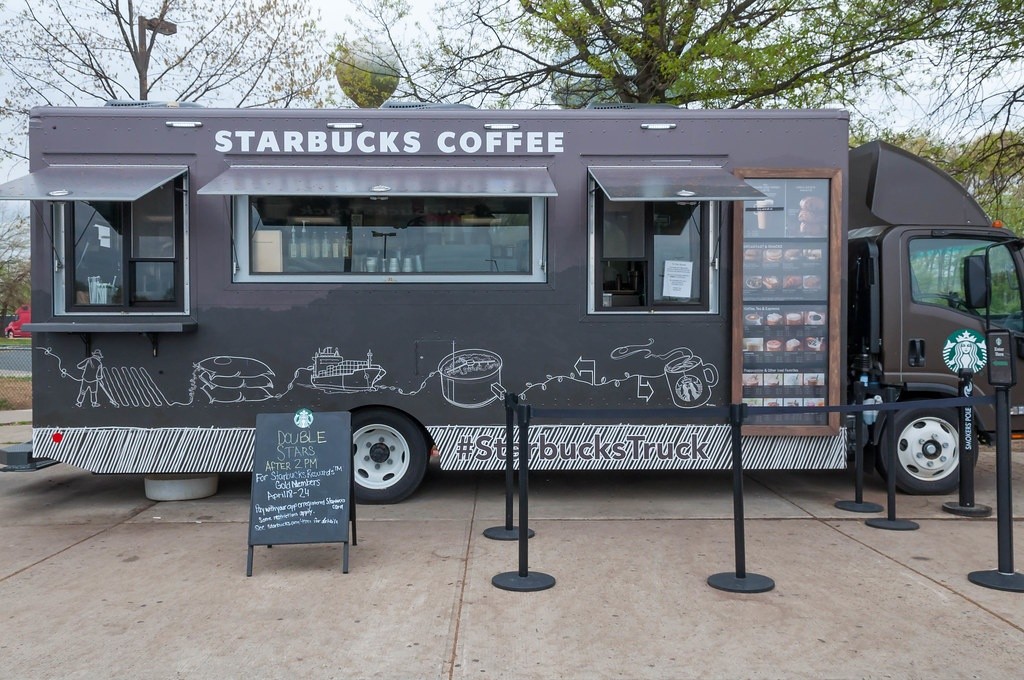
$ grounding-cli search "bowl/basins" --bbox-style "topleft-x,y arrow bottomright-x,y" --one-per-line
746,276 -> 761,289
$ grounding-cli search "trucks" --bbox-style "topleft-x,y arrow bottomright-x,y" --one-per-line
0,102 -> 1024,505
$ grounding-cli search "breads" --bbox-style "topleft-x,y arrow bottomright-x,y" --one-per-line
798,196 -> 823,235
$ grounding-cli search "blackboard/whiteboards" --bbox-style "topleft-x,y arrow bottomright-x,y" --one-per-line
247,411 -> 351,545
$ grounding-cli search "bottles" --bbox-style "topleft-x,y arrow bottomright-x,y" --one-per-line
332,231 -> 341,259
289,229 -> 298,257
342,232 -> 351,259
415,253 -> 424,272
321,231 -> 330,259
615,274 -> 622,289
311,231 -> 320,258
299,229 -> 309,257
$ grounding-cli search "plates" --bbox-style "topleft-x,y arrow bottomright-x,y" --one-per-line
803,275 -> 822,290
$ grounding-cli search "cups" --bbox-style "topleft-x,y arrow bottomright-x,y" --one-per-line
747,403 -> 757,420
767,314 -> 783,325
745,379 -> 759,386
767,378 -> 779,386
403,258 -> 412,272
787,402 -> 798,420
389,258 -> 400,272
807,312 -> 824,325
808,377 -> 818,385
787,313 -> 801,326
753,200 -> 774,229
88,276 -> 100,304
767,340 -> 781,351
745,314 -> 760,325
786,339 -> 800,351
767,402 -> 779,419
505,246 -> 514,257
98,283 -> 113,304
747,341 -> 760,351
366,257 -> 376,272
807,339 -> 820,352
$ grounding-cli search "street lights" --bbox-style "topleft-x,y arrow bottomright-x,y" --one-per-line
136,14 -> 178,100
